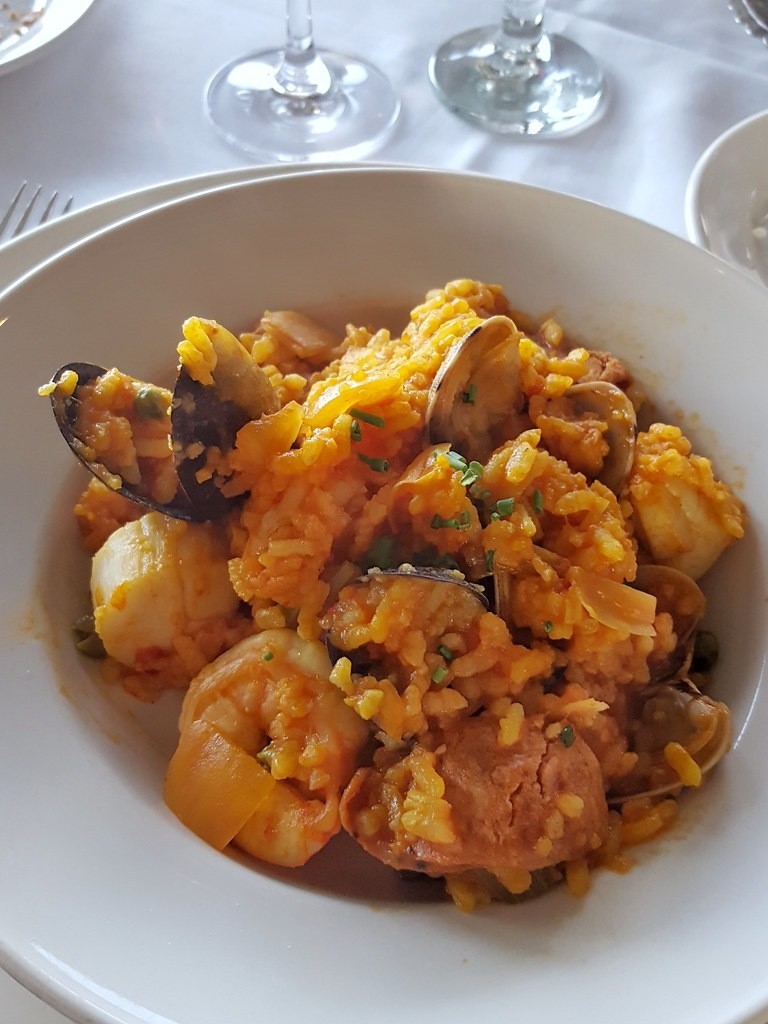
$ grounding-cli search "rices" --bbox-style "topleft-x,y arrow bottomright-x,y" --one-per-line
36,276 -> 744,911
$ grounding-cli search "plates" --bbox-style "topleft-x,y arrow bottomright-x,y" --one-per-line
0,161 -> 374,292
0,0 -> 96,71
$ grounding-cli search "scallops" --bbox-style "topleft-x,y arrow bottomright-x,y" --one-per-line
47,315 -> 732,802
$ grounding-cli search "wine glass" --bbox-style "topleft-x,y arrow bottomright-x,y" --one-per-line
427,0 -> 611,141
203,0 -> 403,163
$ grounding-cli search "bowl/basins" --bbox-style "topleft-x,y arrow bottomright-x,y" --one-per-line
0,167 -> 768,1024
684,110 -> 768,289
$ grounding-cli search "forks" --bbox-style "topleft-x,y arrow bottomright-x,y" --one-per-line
0,180 -> 72,245
